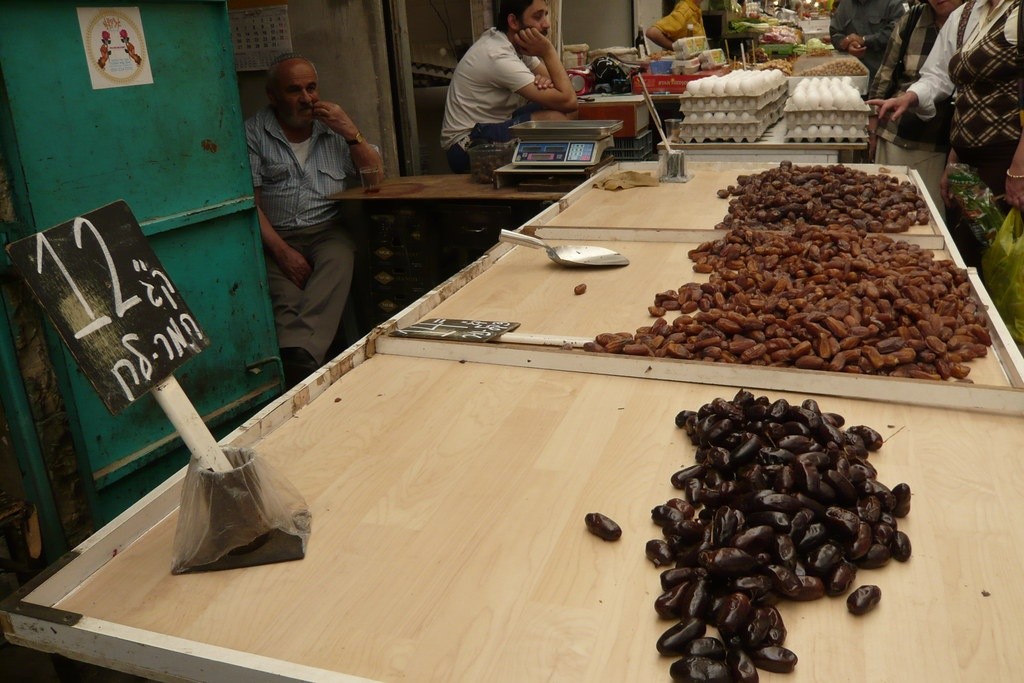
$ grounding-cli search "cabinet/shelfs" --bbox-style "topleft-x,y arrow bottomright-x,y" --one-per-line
702,10 -> 759,49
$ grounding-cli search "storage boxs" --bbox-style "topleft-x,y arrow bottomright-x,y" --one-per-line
649,55 -> 675,75
786,54 -> 870,96
577,96 -> 649,138
467,144 -> 514,185
672,36 -> 708,60
759,43 -> 793,59
698,49 -> 726,70
672,57 -> 701,74
604,130 -> 652,161
632,69 -> 724,96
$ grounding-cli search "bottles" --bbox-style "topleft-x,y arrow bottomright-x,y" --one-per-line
635,25 -> 646,59
686,24 -> 694,38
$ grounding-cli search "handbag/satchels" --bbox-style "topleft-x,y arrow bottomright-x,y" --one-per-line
591,53 -> 632,94
981,206 -> 1024,345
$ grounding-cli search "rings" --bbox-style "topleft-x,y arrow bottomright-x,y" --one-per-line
845,36 -> 849,40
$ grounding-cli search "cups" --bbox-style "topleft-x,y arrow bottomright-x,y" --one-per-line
360,164 -> 380,193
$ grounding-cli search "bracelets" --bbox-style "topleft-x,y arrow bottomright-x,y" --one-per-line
1006,168 -> 1024,179
867,129 -> 877,136
862,34 -> 866,43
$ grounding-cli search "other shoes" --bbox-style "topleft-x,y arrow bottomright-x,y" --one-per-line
280,347 -> 318,379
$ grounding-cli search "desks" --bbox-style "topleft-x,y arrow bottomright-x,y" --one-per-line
656,139 -> 868,164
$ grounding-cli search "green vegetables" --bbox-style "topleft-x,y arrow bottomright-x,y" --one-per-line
727,18 -> 778,33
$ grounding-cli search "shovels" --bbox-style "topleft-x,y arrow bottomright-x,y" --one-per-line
497,228 -> 630,269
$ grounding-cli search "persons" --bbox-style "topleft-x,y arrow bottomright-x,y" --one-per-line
243,52 -> 387,389
829,0 -> 1024,324
645,0 -> 710,52
439,0 -> 579,174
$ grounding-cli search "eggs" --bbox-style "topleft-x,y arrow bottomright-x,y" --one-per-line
684,68 -> 861,136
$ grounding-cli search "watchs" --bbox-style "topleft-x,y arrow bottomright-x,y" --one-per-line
346,131 -> 364,146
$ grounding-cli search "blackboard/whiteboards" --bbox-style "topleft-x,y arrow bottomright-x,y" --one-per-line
1,198 -> 212,423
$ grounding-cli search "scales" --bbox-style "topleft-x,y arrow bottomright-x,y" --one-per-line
506,118 -> 623,168
